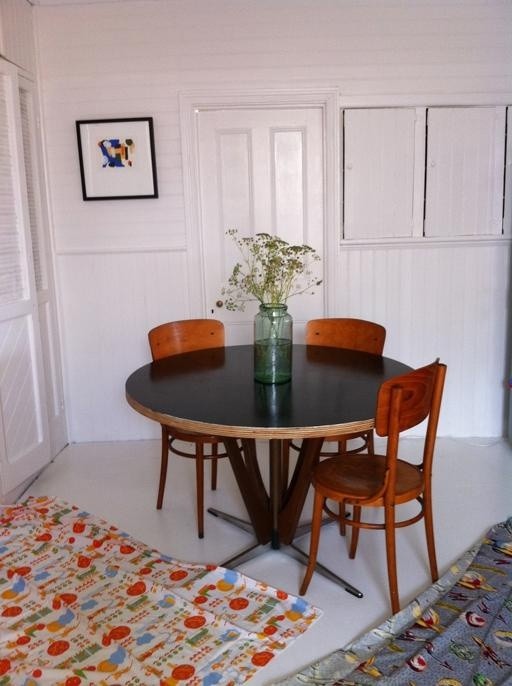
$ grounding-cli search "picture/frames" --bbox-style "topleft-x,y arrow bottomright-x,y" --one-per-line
74,116 -> 160,206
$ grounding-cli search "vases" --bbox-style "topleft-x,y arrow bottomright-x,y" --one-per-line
251,303 -> 295,387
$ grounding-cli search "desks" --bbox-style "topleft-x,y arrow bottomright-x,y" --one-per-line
127,344 -> 414,598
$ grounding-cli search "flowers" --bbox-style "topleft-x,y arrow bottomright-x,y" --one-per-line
202,222 -> 323,381
